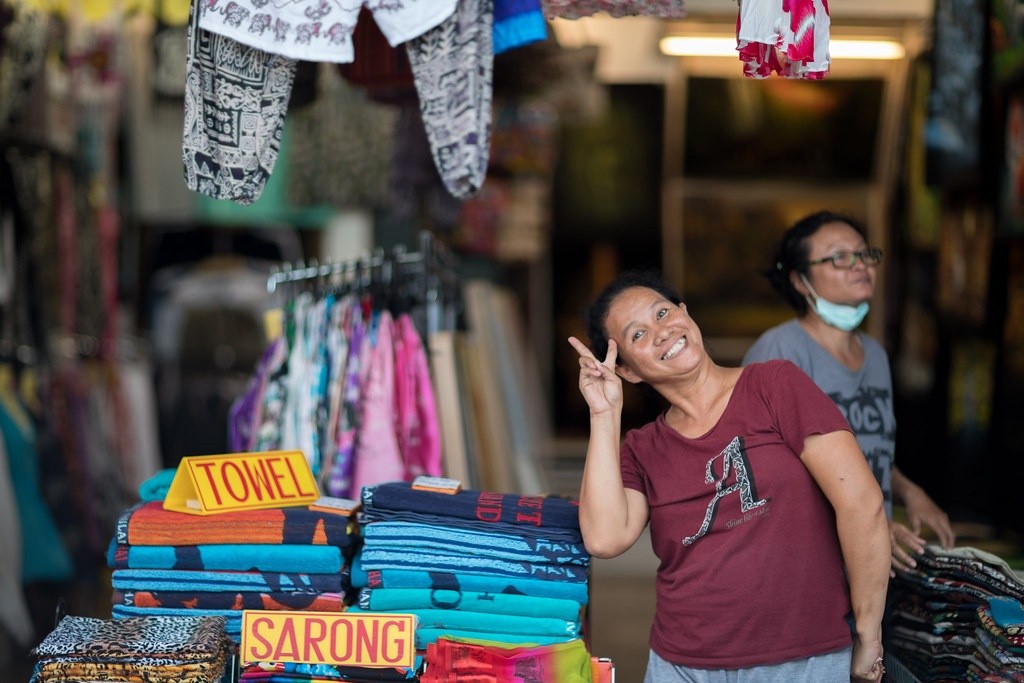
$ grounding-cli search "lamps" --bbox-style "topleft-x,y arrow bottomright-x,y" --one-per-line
659,19 -> 907,62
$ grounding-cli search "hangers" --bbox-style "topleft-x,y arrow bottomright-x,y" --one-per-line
266,245 -> 414,349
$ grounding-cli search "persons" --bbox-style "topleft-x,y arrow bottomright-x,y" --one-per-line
735,209 -> 956,683
567,267 -> 892,683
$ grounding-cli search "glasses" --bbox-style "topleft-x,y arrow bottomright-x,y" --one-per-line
809,246 -> 882,268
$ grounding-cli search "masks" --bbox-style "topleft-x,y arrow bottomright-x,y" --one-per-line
800,274 -> 871,331
